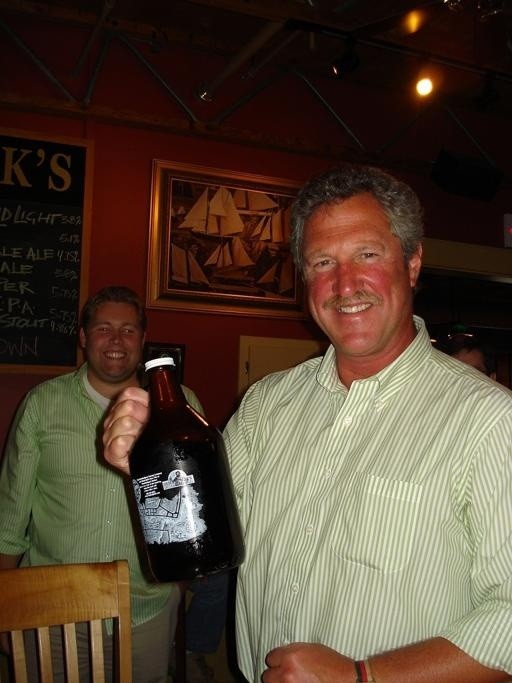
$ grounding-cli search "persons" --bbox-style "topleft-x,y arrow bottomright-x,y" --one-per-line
102,163 -> 512,683
444,339 -> 498,382
1,286 -> 203,682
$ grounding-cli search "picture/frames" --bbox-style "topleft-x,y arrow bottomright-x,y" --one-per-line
143,157 -> 314,321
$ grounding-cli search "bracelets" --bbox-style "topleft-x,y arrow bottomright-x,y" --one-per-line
354,659 -> 376,683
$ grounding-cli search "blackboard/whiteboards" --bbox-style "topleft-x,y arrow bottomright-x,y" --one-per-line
0,128 -> 95,375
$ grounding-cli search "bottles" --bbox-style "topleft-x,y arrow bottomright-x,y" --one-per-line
124,352 -> 247,588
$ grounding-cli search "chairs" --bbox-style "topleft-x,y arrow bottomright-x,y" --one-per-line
2,559 -> 134,682
